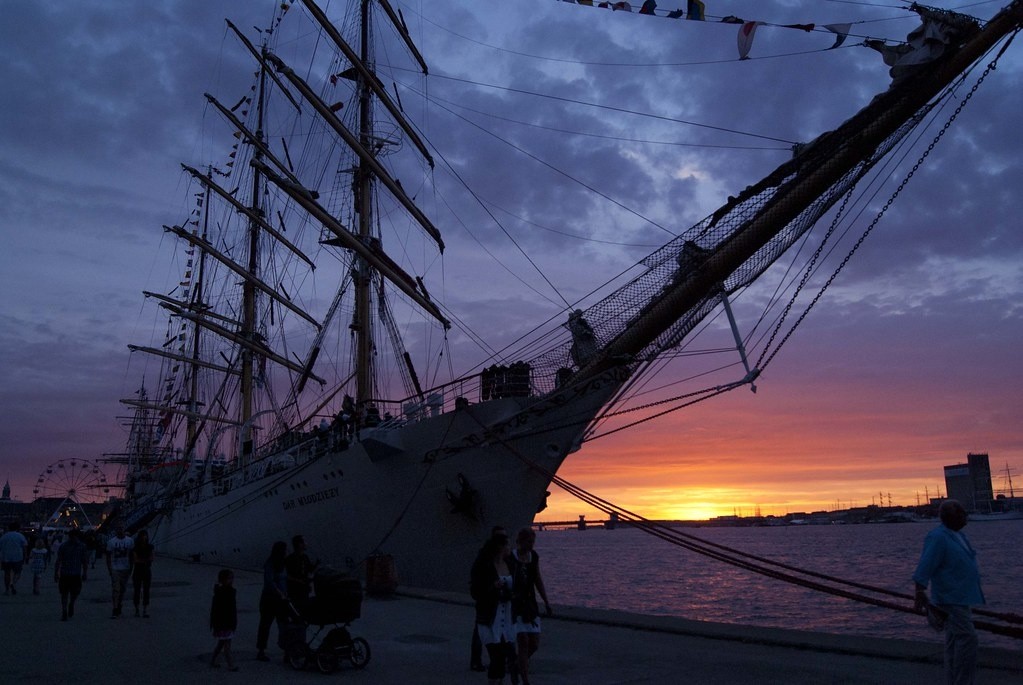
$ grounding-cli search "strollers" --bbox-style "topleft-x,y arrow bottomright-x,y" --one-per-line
272,574 -> 372,674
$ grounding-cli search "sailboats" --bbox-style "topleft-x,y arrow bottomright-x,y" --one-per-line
34,0 -> 1023,580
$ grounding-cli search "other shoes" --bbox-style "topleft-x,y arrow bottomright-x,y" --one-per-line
143,613 -> 148,617
470,663 -> 485,672
118,603 -> 122,614
257,652 -> 270,662
136,612 -> 140,616
210,661 -> 220,668
227,665 -> 238,671
11,584 -> 16,594
112,608 -> 119,617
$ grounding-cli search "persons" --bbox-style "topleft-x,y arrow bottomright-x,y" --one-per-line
211,569 -> 239,672
313,403 -> 379,456
256,535 -> 323,664
0,521 -> 155,621
912,499 -> 986,685
470,525 -> 553,685
